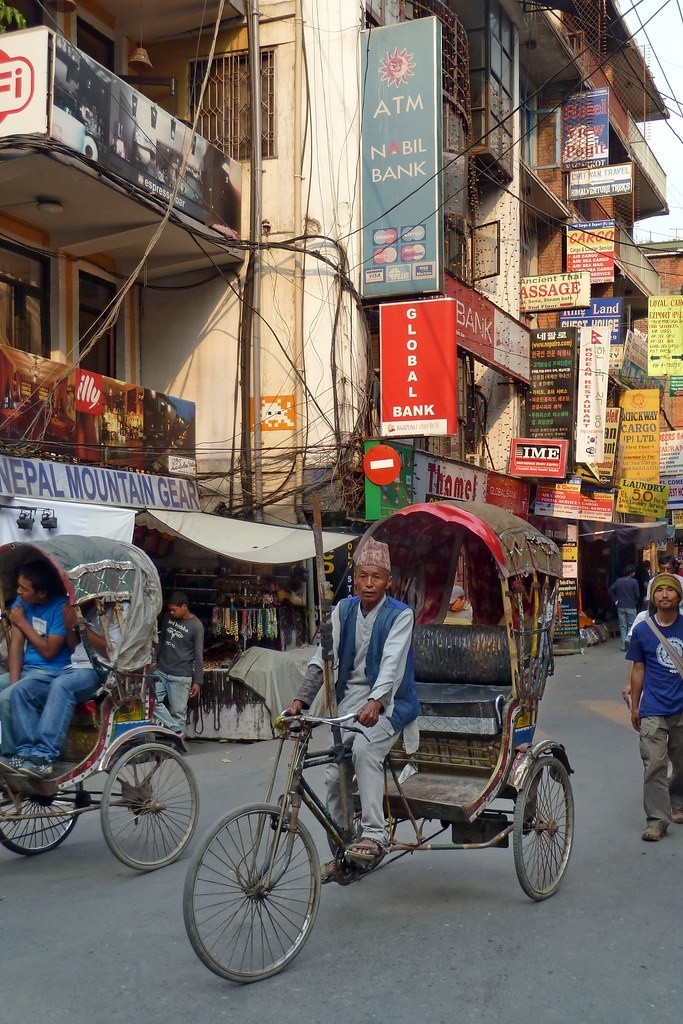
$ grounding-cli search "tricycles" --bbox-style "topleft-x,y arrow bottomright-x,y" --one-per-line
181,501 -> 576,985
0,533 -> 200,873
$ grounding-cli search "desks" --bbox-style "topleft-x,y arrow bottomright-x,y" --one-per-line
185,660 -> 274,740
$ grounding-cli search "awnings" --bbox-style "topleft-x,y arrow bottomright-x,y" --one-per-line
579,519 -> 667,550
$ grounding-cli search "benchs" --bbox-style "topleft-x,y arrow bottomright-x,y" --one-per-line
62,682 -> 144,760
408,623 -> 532,741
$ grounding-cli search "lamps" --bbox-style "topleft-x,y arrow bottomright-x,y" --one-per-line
41,508 -> 57,531
47,0 -> 77,13
37,200 -> 63,215
128,0 -> 153,71
17,508 -> 35,531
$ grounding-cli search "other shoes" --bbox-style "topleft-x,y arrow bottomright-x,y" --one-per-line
621,648 -> 626,651
174,731 -> 188,753
168,743 -> 184,758
642,826 -> 666,840
672,809 -> 683,823
624,639 -> 630,648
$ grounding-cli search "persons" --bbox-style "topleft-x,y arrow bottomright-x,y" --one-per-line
625,573 -> 683,842
1,560 -> 130,777
446,585 -> 473,621
151,590 -> 204,755
286,536 -> 420,880
607,553 -> 683,710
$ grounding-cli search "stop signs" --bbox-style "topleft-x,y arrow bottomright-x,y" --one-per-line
364,446 -> 402,485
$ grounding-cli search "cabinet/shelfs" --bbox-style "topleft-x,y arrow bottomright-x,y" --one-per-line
157,572 -> 306,607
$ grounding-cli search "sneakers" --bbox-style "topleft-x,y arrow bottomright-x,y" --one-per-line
0,755 -> 33,774
18,755 -> 53,778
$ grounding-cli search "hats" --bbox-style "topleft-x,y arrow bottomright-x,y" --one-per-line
449,586 -> 464,604
356,536 -> 391,573
650,574 -> 683,605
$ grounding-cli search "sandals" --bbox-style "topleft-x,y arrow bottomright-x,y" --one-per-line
344,845 -> 380,867
320,859 -> 349,884
